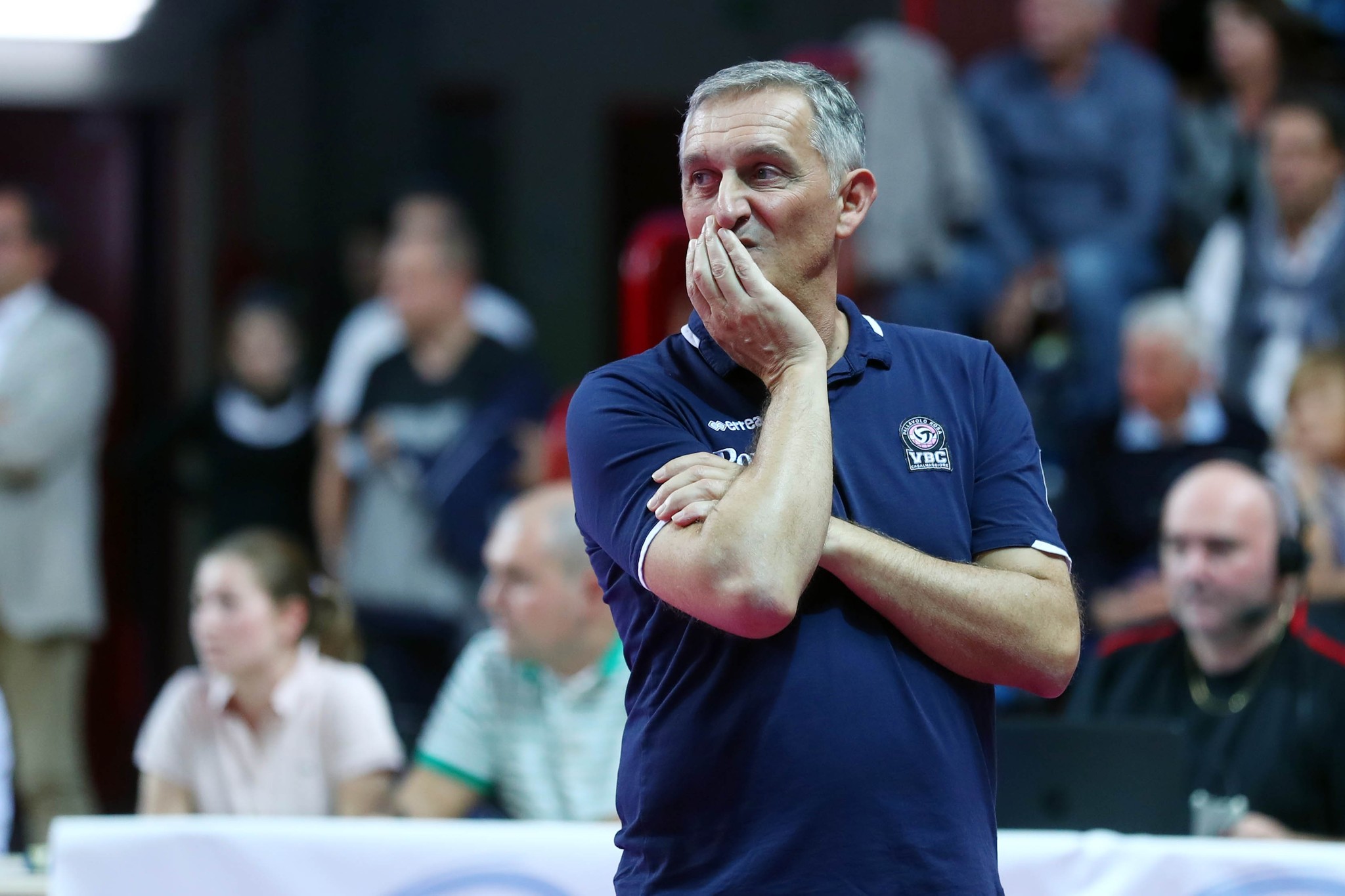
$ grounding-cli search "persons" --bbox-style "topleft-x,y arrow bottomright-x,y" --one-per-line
131,187 -> 631,836
1,180 -> 111,854
793,0 -> 1345,841
564,61 -> 1084,896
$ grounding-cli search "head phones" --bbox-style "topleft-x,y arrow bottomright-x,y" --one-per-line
1256,465 -> 1312,576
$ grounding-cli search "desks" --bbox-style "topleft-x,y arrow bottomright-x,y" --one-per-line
45,812 -> 1345,896
